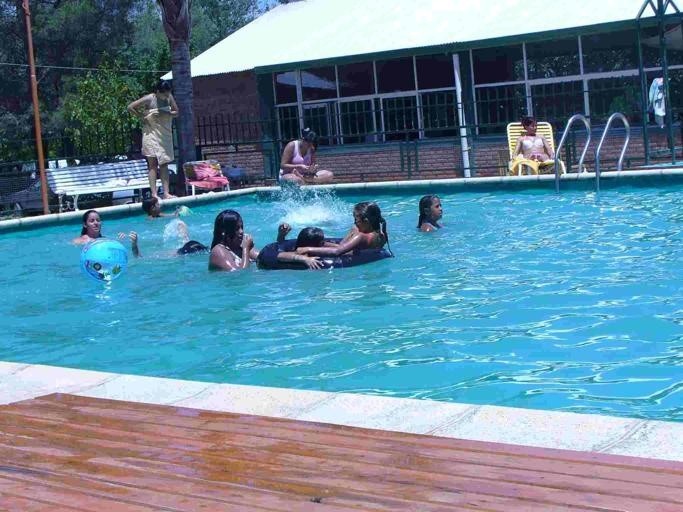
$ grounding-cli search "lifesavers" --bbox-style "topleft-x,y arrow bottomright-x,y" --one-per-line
255,237 -> 393,270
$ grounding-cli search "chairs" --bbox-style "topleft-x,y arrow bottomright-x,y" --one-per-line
183,159 -> 231,195
507,122 -> 567,175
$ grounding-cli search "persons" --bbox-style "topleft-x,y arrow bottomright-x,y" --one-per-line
206,208 -> 291,272
276,127 -> 334,187
139,196 -> 180,219
509,115 -> 556,163
128,219 -> 208,260
274,226 -> 356,271
414,193 -> 445,232
71,209 -> 126,245
127,79 -> 183,198
294,200 -> 393,258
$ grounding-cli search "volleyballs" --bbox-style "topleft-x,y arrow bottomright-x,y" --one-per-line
80,238 -> 128,281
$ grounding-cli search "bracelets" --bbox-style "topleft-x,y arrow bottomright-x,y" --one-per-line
294,163 -> 297,169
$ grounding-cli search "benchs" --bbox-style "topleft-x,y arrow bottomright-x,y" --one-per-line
43,159 -> 163,212
